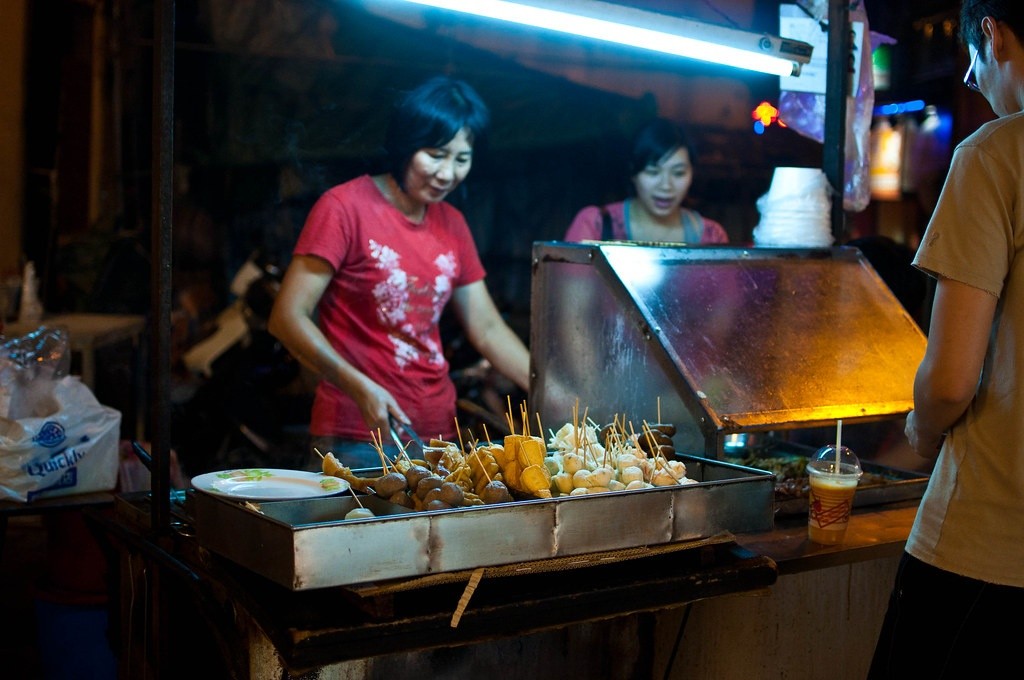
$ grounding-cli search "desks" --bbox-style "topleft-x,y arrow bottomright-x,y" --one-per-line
3,314 -> 147,392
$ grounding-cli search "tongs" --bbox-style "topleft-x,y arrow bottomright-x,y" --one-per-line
389,413 -> 425,466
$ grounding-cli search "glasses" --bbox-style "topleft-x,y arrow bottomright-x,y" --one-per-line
962,49 -> 985,94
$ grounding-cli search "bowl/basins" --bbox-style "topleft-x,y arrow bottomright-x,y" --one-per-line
757,165 -> 834,248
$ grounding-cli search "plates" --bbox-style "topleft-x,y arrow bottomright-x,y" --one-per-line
191,469 -> 351,501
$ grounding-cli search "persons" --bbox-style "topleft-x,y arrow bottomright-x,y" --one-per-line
864,0 -> 1024,680
37,202 -> 515,479
269,78 -> 532,471
796,233 -> 939,473
565,118 -> 768,445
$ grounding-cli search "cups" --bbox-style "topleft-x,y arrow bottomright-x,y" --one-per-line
807,445 -> 862,545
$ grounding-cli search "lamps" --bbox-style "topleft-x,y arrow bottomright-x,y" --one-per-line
404,0 -> 814,77
